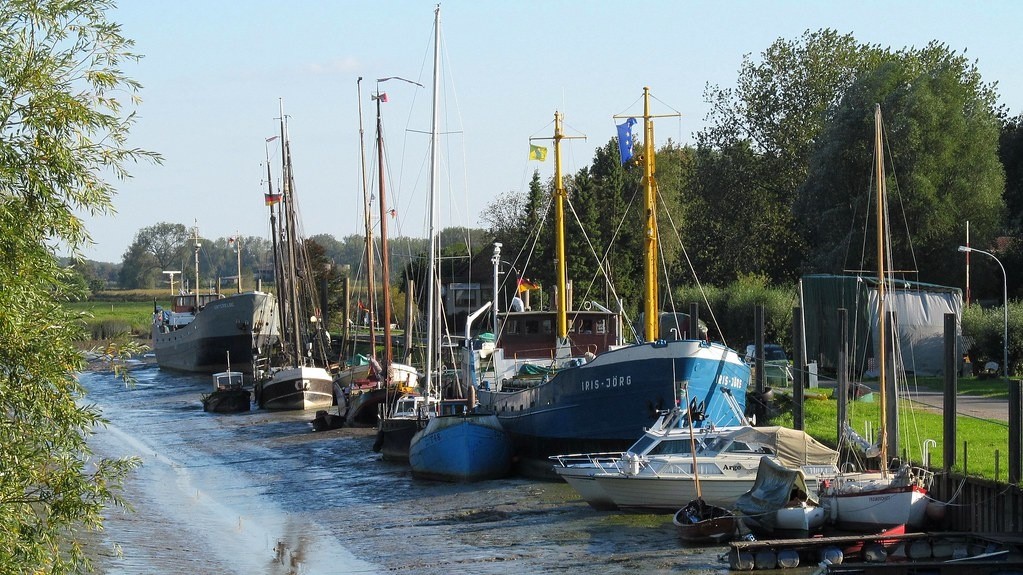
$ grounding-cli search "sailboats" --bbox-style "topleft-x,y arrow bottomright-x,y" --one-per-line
254,2 -> 934,543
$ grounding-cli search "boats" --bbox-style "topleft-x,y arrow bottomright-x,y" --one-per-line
199,348 -> 254,415
145,223 -> 292,376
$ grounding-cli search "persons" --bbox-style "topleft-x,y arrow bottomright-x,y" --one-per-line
580,320 -> 592,333
516,321 -> 538,334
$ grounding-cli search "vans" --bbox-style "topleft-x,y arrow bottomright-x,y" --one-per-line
745,344 -> 788,362
172,293 -> 226,314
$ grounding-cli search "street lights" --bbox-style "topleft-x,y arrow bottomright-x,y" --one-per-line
958,246 -> 1009,379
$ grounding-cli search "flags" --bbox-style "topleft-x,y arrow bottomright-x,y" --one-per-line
529,145 -> 547,162
258,193 -> 282,206
518,275 -> 541,292
616,117 -> 637,165
